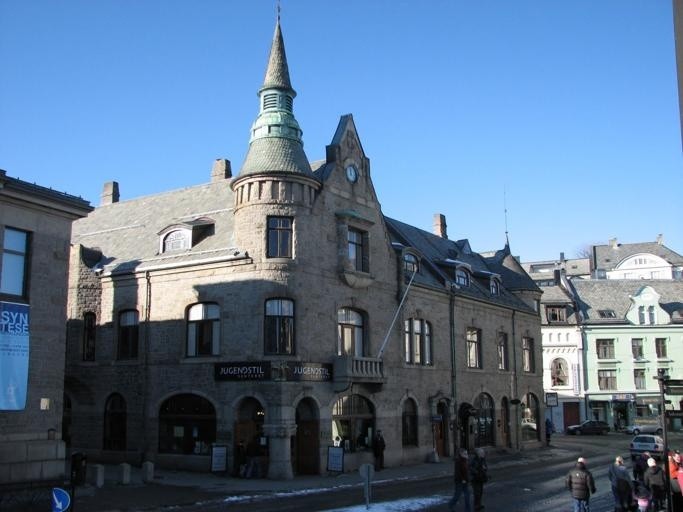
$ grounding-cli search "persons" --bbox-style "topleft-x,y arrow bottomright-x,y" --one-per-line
564,445 -> 682,512
235,441 -> 267,479
368,428 -> 386,473
446,448 -> 471,512
545,417 -> 552,446
468,447 -> 489,511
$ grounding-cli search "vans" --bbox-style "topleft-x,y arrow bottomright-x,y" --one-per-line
622,416 -> 663,435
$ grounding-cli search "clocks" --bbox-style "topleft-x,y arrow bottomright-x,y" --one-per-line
345,165 -> 357,182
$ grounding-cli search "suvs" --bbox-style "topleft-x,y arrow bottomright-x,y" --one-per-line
567,420 -> 610,436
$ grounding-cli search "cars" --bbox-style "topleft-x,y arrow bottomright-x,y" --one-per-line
630,434 -> 664,460
521,418 -> 537,430
